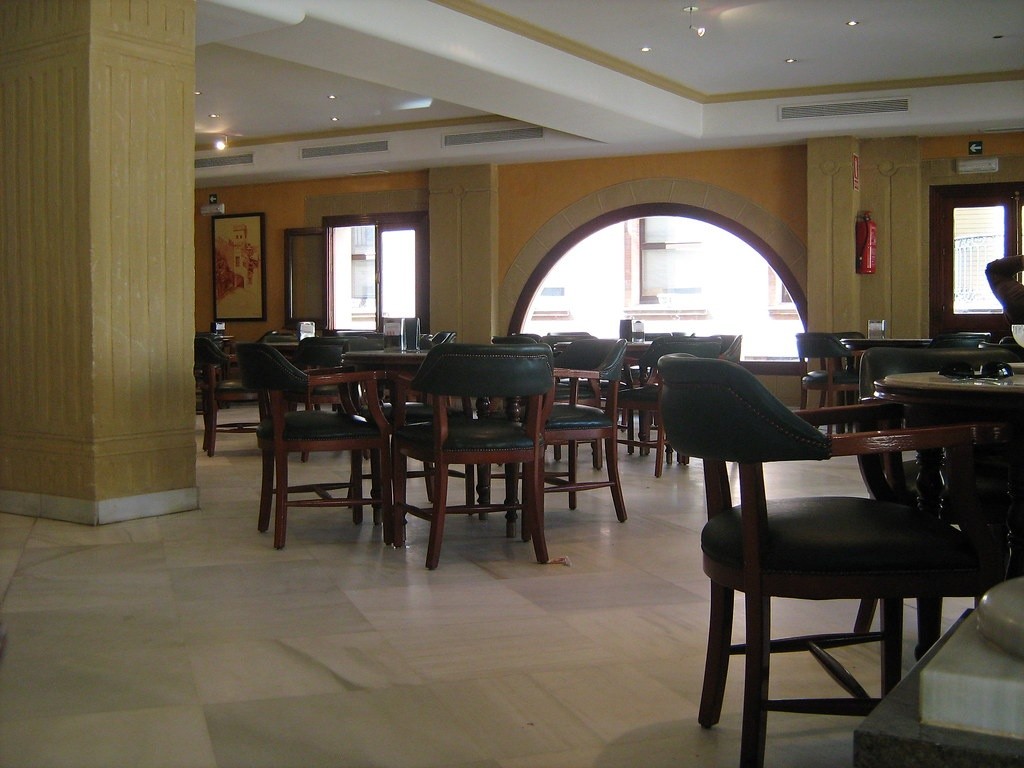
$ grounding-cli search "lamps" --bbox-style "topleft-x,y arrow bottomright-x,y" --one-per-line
955,158 -> 998,174
201,203 -> 224,216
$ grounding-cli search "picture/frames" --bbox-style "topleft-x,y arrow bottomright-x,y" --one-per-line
211,212 -> 267,321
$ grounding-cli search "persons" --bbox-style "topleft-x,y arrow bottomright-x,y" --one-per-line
985,255 -> 1024,325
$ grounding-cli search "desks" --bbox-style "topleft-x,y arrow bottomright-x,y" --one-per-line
874,370 -> 1024,535
625,341 -> 653,454
264,342 -> 320,411
853,609 -> 1024,768
840,338 -> 931,434
341,350 -> 428,540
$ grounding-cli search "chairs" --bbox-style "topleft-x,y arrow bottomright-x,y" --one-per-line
194,329 -> 1024,768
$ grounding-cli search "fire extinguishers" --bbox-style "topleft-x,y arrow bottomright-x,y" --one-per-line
855,210 -> 876,275
